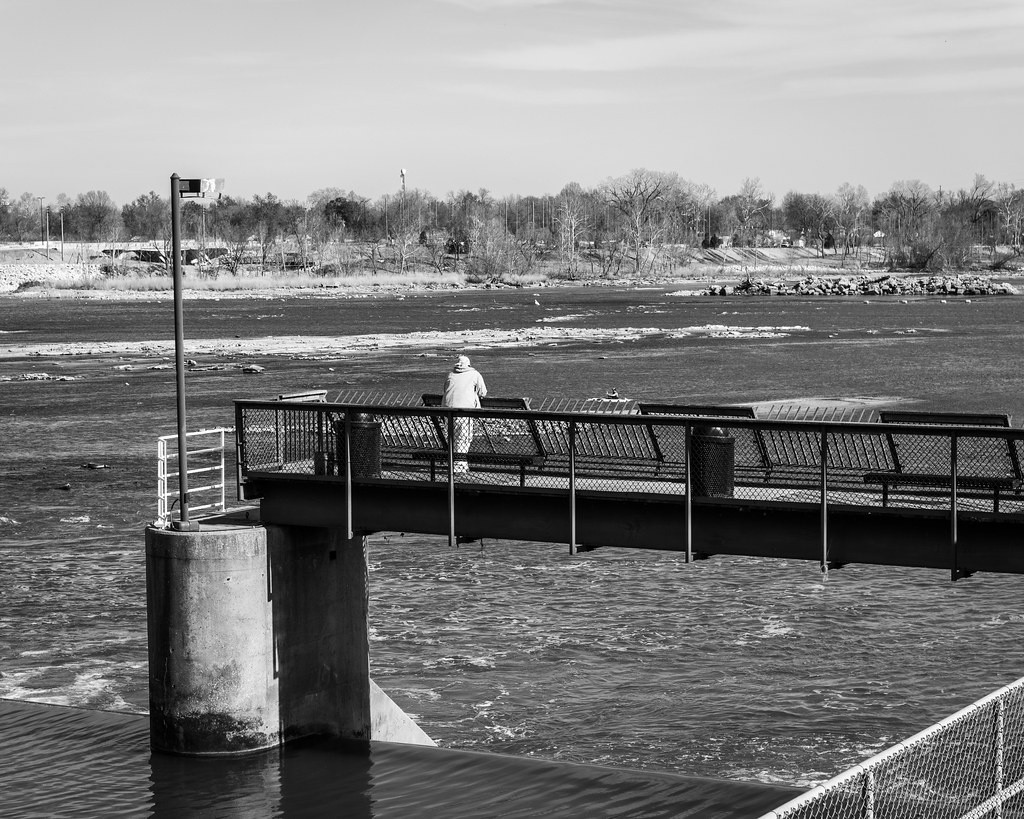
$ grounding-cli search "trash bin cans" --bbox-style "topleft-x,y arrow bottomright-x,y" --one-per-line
692,424 -> 735,497
333,412 -> 382,476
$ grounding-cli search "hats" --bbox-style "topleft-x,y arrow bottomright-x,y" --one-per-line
457,355 -> 470,366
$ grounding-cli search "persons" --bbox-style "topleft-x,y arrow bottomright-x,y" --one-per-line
442,355 -> 487,473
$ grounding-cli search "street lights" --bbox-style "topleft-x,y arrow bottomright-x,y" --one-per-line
169,172 -> 227,533
